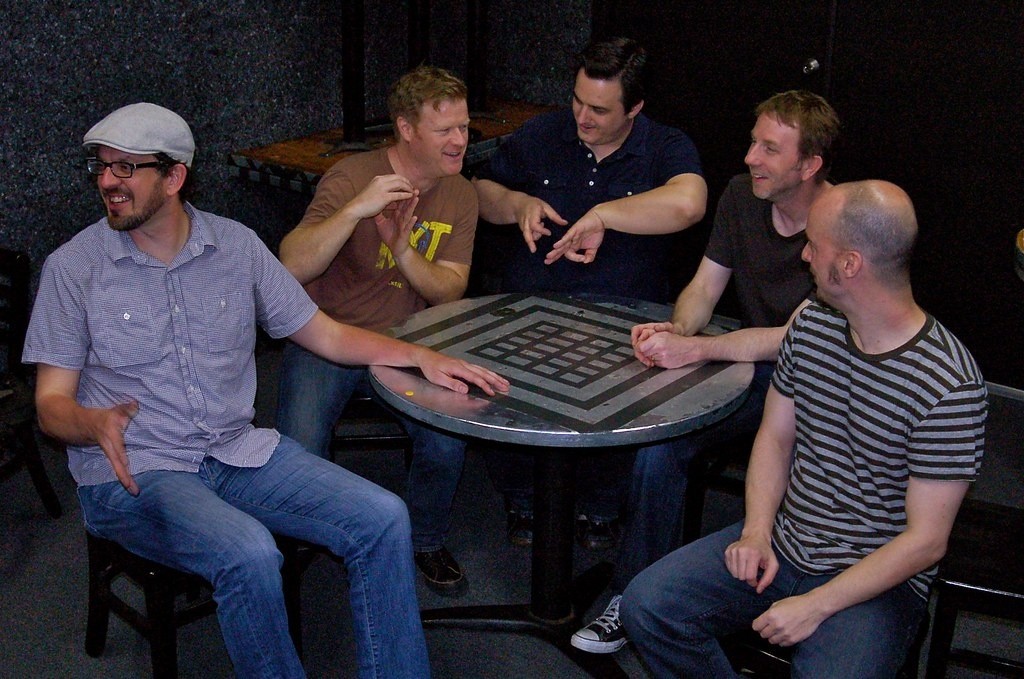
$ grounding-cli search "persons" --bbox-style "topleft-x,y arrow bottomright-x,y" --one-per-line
468,33 -> 708,548
568,87 -> 837,656
270,65 -> 479,595
622,179 -> 991,679
18,101 -> 511,679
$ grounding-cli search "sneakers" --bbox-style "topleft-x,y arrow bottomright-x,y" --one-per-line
571,594 -> 632,654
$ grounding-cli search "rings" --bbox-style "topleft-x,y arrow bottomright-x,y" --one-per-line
651,356 -> 655,361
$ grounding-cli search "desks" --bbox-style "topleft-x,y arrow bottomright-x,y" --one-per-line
226,95 -> 550,199
369,291 -> 757,679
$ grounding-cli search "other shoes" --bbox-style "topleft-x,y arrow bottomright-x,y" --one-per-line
574,517 -> 620,551
508,501 -> 534,547
414,547 -> 466,594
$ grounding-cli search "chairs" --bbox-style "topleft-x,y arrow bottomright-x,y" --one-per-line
83,530 -> 318,679
328,396 -> 412,471
925,378 -> 1024,679
684,429 -> 754,547
730,628 -> 792,679
0,383 -> 62,519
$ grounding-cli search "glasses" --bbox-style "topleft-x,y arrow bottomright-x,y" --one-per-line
85,156 -> 172,178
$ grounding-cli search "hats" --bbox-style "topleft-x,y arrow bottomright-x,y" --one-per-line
82,102 -> 195,171
